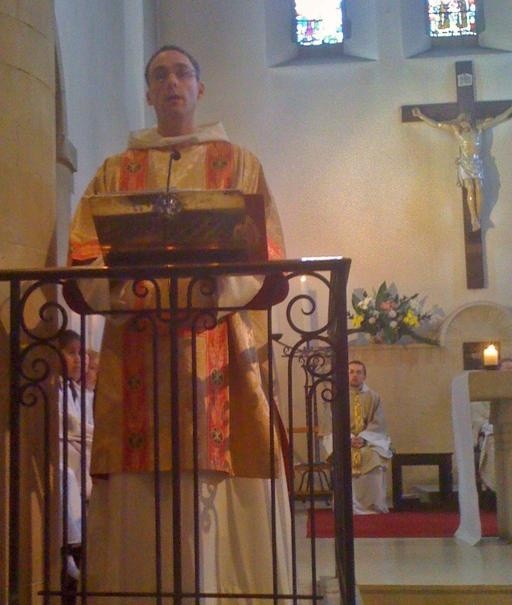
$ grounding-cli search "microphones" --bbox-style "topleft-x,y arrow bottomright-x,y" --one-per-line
166,150 -> 182,193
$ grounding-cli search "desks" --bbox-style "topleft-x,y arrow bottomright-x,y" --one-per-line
391,452 -> 453,510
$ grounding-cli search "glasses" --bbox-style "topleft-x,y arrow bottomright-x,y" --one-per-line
149,69 -> 197,82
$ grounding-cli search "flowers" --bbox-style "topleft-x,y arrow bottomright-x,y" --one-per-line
346,282 -> 438,346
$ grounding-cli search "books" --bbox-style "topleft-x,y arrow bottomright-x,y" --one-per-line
84,190 -> 249,280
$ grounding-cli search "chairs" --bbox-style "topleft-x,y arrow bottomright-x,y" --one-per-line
287,425 -> 335,512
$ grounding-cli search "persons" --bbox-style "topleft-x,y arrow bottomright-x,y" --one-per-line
316,359 -> 394,518
472,357 -> 511,512
57,328 -> 100,580
410,102 -> 511,233
63,43 -> 298,603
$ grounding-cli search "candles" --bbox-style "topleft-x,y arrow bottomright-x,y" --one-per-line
483,344 -> 498,366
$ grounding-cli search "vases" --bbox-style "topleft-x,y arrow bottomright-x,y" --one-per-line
372,334 -> 398,345
